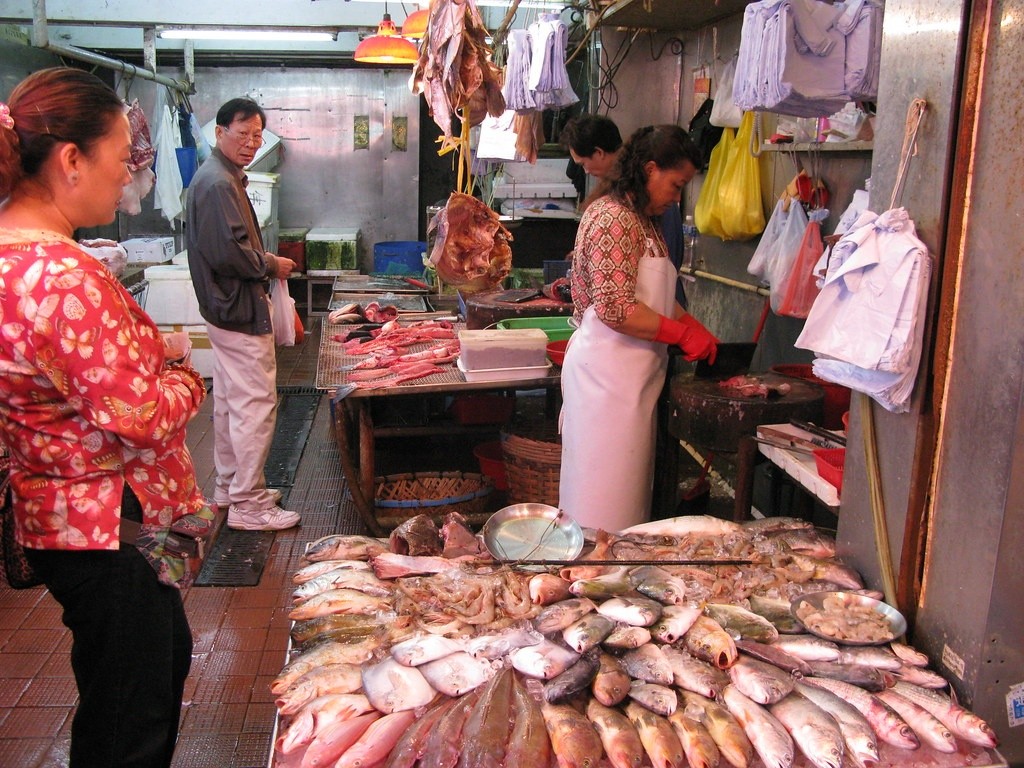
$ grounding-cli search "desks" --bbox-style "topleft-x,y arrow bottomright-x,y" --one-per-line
328,365 -> 563,536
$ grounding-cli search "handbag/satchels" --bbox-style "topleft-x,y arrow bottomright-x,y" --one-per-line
0,440 -> 45,589
709,51 -> 743,128
119,495 -> 219,589
748,199 -> 825,318
694,109 -> 768,240
270,277 -> 303,348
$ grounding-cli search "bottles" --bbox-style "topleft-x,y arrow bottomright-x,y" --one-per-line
681,215 -> 698,269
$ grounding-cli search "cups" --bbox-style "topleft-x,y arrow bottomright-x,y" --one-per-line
796,116 -> 817,142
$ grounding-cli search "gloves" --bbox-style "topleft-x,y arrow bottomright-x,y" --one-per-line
678,312 -> 720,344
649,314 -> 717,366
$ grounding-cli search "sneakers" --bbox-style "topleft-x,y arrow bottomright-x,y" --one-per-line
226,503 -> 302,531
214,489 -> 283,509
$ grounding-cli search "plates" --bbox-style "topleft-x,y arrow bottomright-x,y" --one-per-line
791,593 -> 907,646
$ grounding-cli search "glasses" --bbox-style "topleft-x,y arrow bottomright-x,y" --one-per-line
223,127 -> 266,148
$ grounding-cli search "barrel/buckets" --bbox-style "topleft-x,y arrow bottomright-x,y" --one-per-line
374,241 -> 429,275
768,365 -> 852,431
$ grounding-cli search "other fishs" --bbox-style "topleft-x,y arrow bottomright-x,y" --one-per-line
277,510 -> 1002,768
326,302 -> 462,404
408,0 -> 507,154
426,189 -> 513,291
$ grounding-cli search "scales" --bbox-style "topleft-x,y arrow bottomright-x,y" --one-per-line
464,502 -> 756,568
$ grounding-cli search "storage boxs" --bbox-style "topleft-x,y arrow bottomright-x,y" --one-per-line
156,323 -> 214,379
456,357 -> 554,383
450,393 -> 521,428
120,236 -> 175,264
241,170 -> 282,226
458,327 -> 551,369
756,421 -> 848,540
136,265 -> 207,325
259,220 -> 279,256
496,315 -> 577,344
278,227 -> 313,273
305,227 -> 362,269
199,117 -> 281,173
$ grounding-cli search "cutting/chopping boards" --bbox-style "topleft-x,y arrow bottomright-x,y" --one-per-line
670,369 -> 824,452
466,289 -> 574,330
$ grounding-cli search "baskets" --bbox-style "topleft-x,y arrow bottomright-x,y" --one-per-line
348,470 -> 495,530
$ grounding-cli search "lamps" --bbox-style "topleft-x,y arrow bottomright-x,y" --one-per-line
352,0 -> 421,65
402,0 -> 430,38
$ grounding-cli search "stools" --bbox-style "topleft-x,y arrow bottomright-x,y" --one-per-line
307,271 -> 361,317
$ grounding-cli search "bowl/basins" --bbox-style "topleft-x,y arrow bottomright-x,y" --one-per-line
473,442 -> 508,491
546,340 -> 570,366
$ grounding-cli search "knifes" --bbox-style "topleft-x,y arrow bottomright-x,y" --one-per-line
493,287 -> 543,302
668,342 -> 757,381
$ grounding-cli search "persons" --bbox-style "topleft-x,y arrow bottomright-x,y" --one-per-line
185,99 -> 302,532
559,125 -> 720,537
0,66 -> 219,768
565,116 -> 686,508
